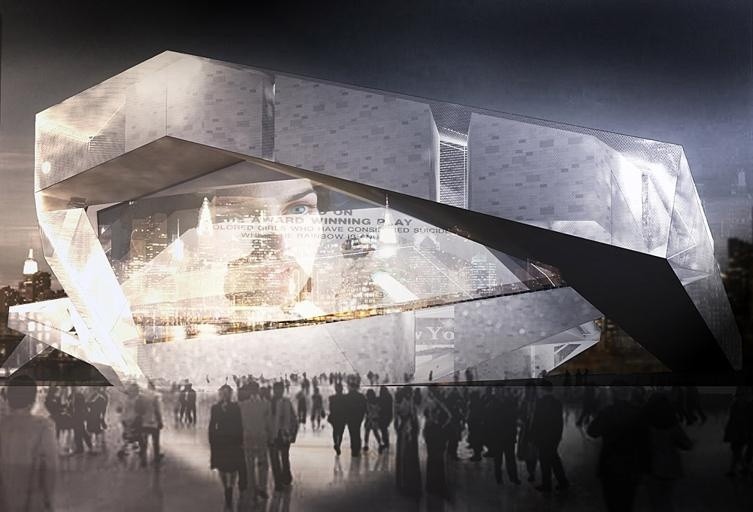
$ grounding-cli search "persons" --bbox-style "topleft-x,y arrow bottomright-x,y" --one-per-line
0,368 -> 752,511
198,177 -> 329,306
